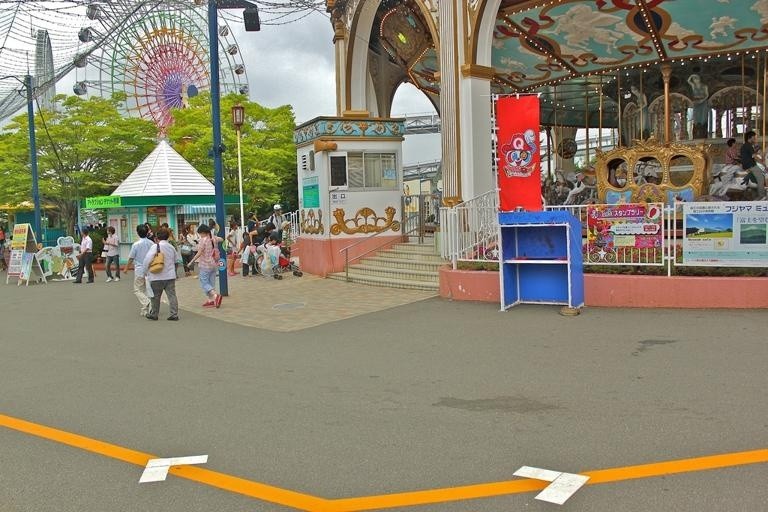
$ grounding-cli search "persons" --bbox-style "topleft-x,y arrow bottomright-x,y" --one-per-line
186,223 -> 224,310
740,130 -> 767,198
85,220 -> 108,256
140,202 -> 292,284
72,228 -> 94,284
0,226 -> 5,242
122,223 -> 155,314
101,226 -> 120,283
142,228 -> 180,321
723,138 -> 742,165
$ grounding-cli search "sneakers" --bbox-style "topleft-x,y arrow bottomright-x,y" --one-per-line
87,281 -> 94,283
168,315 -> 178,320
106,277 -> 113,283
146,314 -> 158,320
215,295 -> 222,306
114,277 -> 120,281
72,279 -> 81,283
202,300 -> 215,307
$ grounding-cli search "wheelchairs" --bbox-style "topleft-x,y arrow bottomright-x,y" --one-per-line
250,243 -> 273,278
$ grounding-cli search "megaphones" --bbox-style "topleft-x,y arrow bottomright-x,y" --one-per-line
314,140 -> 337,153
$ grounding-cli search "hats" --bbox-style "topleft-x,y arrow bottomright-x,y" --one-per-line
274,205 -> 281,211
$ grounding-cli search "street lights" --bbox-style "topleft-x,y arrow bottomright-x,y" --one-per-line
208,1 -> 261,297
228,102 -> 249,234
419,177 -> 438,197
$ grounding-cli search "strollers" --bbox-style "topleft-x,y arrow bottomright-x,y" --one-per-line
272,255 -> 301,281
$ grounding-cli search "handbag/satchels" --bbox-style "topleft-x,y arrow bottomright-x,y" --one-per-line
213,250 -> 221,259
149,253 -> 164,273
